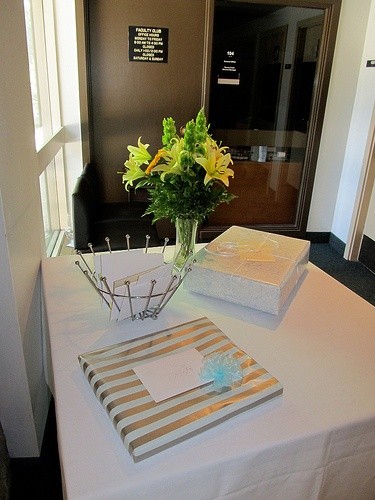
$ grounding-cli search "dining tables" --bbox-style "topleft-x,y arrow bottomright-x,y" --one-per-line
41,243 -> 375,500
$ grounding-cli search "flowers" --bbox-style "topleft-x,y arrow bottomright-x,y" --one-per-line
117,104 -> 240,258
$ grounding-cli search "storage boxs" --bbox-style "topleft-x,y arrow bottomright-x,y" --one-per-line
183,226 -> 311,314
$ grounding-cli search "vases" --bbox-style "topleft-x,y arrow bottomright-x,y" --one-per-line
173,219 -> 198,269
